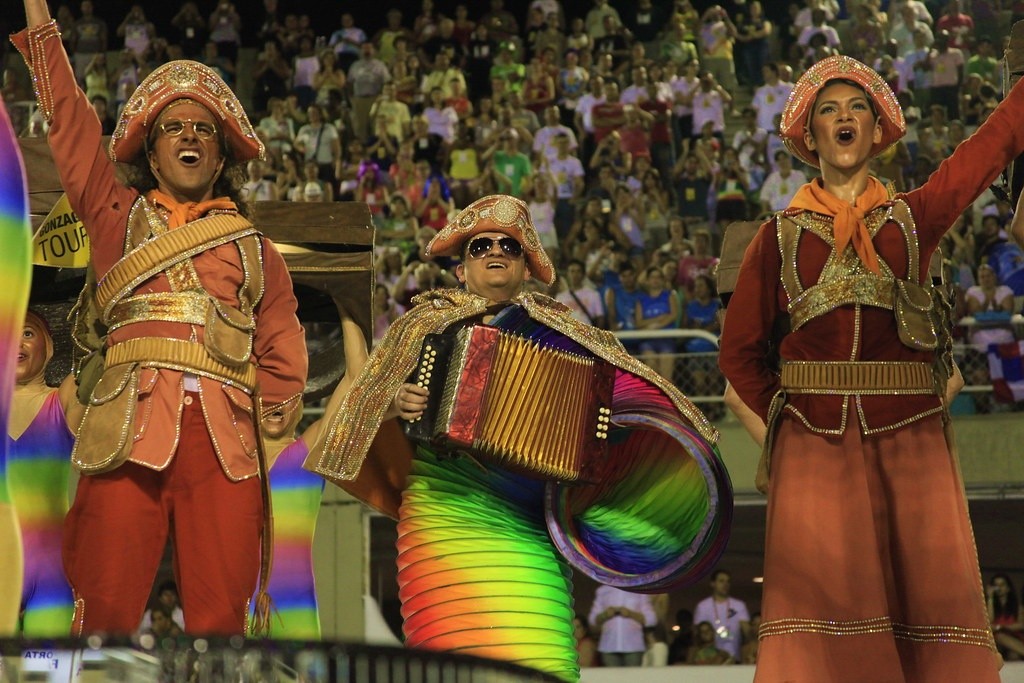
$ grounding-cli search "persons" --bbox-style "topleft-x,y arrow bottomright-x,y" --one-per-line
10,1 -> 309,639
342,266 -> 458,384
983,576 -> 1024,661
720,56 -> 1024,683
303,196 -> 719,683
6,314 -> 87,639
574,569 -> 760,667
138,585 -> 185,636
0,0 -> 1023,416
724,356 -> 964,447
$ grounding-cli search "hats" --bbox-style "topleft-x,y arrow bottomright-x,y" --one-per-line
779,55 -> 906,169
108,60 -> 266,169
424,194 -> 557,288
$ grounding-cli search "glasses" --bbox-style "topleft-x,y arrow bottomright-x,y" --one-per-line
464,237 -> 525,259
159,117 -> 218,139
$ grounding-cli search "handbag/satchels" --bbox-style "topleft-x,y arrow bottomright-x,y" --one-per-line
591,315 -> 612,333
